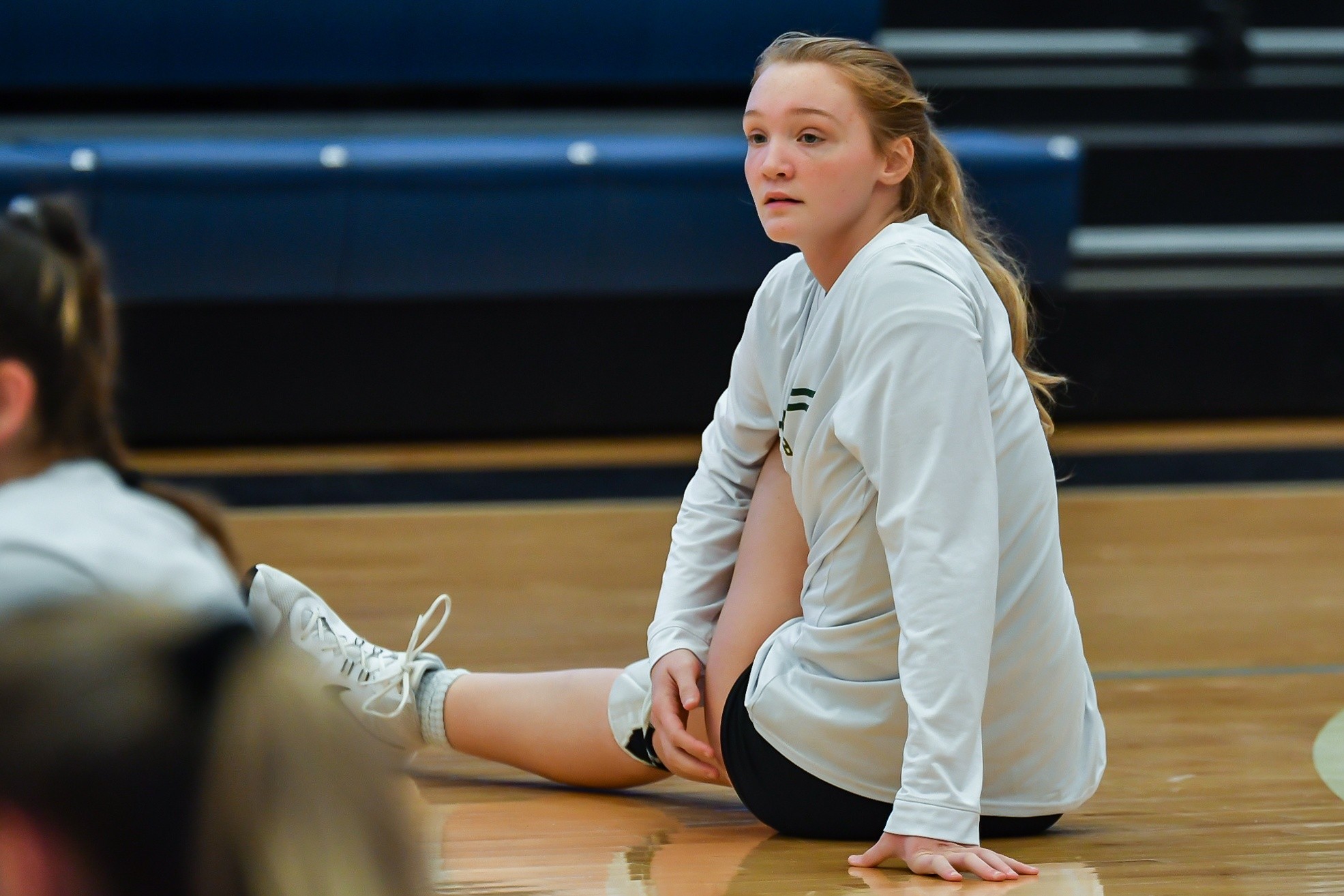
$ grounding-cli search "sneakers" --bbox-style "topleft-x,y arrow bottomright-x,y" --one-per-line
237,561 -> 452,750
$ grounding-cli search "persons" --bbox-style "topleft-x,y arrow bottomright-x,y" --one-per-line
241,33 -> 1107,881
0,187 -> 437,896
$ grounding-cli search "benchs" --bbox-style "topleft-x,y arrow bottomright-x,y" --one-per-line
0,0 -> 1080,304
